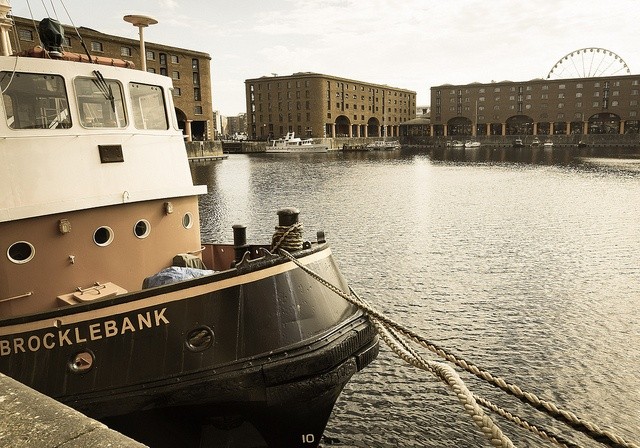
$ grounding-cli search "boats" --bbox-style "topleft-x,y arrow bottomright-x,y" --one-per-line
383,140 -> 399,148
0,0 -> 380,448
465,140 -> 480,147
515,138 -> 522,146
452,139 -> 463,146
544,140 -> 554,145
264,138 -> 328,153
530,137 -> 540,146
578,140 -> 585,145
